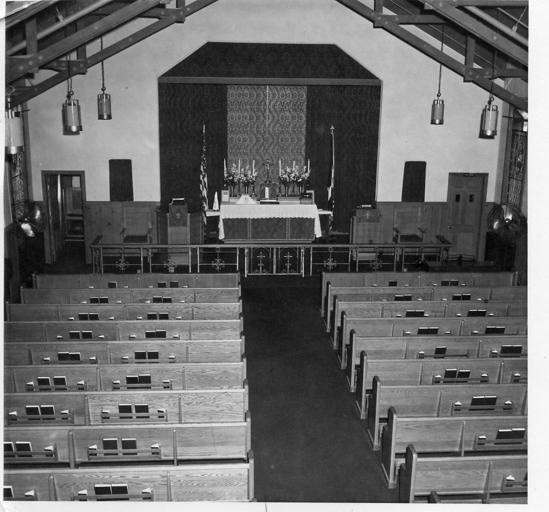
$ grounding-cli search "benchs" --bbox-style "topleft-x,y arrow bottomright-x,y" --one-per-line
4,266 -> 261,501
316,268 -> 528,501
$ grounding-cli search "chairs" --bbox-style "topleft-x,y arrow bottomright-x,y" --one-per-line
392,205 -> 428,256
119,206 -> 153,258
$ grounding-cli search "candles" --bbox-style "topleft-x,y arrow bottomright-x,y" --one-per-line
219,158 -> 316,182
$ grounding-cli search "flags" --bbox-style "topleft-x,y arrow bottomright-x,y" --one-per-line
327,133 -> 336,202
200,133 -> 208,225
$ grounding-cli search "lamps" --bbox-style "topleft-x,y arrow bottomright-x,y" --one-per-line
479,30 -> 502,141
63,40 -> 87,136
428,17 -> 449,128
95,36 -> 115,121
6,93 -> 26,156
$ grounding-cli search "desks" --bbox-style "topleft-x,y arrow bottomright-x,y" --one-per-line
218,199 -> 319,244
